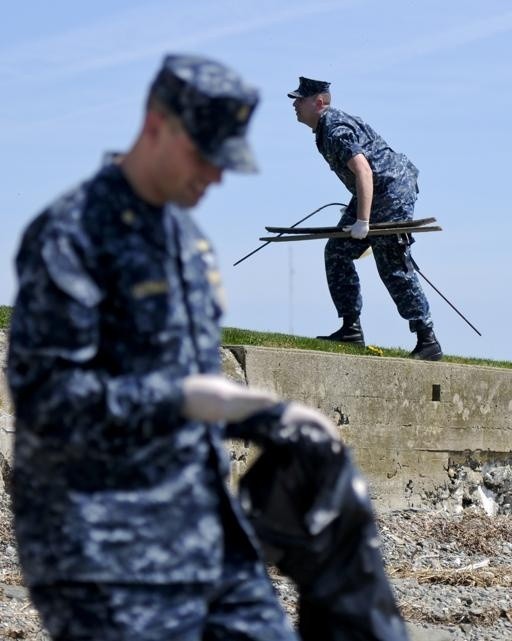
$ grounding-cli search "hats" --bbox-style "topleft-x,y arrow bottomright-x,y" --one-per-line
286,76 -> 332,100
148,55 -> 262,175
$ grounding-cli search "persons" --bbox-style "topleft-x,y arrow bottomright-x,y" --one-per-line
6,49 -> 345,641
287,76 -> 444,364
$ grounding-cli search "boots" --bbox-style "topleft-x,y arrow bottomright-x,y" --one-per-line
315,315 -> 365,346
406,326 -> 443,361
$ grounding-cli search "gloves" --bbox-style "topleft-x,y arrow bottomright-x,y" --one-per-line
179,376 -> 279,424
341,218 -> 370,240
275,401 -> 341,454
